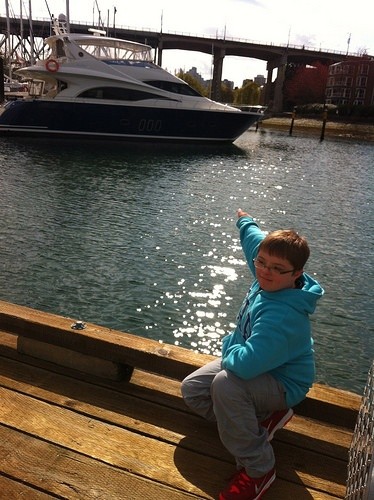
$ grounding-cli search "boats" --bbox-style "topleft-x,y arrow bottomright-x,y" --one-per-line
0,14 -> 268,143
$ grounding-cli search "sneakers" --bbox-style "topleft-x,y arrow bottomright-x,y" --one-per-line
259,407 -> 296,443
217,466 -> 277,500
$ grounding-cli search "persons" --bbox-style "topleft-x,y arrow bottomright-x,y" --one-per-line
180,208 -> 325,500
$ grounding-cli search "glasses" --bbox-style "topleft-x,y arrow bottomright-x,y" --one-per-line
252,258 -> 299,275
